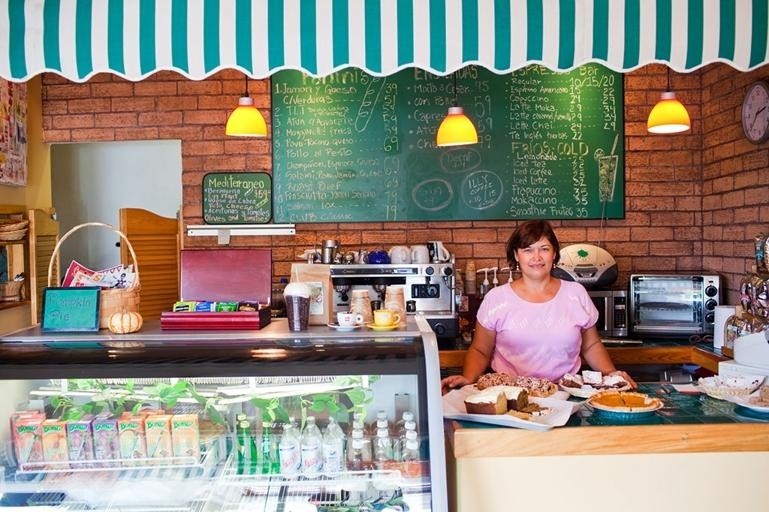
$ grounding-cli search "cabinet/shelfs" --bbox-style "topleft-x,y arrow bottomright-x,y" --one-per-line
1,210 -> 30,336
1,310 -> 452,512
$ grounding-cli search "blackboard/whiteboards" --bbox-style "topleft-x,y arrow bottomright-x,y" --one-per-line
202,172 -> 273,224
270,63 -> 626,224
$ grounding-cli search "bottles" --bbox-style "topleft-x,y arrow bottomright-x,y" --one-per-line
236,408 -> 418,476
466,260 -> 478,296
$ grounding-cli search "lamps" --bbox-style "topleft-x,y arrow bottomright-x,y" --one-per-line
223,69 -> 270,139
436,70 -> 481,150
645,63 -> 694,133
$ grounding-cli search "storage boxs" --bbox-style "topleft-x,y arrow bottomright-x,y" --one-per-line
158,245 -> 274,331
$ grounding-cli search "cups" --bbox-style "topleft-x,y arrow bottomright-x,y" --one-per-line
373,309 -> 401,325
350,289 -> 372,324
307,238 -> 450,265
335,311 -> 363,325
285,296 -> 310,331
385,286 -> 410,327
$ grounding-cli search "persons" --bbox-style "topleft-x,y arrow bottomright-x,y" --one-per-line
441,220 -> 638,396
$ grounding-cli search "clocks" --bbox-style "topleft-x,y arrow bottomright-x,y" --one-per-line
739,80 -> 769,145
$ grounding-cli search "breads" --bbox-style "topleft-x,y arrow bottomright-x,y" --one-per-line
464,371 -> 661,421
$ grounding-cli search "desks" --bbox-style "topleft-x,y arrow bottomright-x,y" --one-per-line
695,337 -> 736,376
433,340 -> 695,369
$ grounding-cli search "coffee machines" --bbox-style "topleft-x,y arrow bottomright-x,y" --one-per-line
327,264 -> 458,337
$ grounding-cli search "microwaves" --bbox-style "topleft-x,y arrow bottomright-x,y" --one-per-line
587,289 -> 628,337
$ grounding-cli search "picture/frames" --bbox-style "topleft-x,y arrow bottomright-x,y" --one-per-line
40,286 -> 103,333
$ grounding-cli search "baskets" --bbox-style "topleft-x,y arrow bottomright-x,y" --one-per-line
46,220 -> 143,331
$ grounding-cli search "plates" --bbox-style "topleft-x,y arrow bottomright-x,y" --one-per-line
327,322 -> 362,332
734,406 -> 769,423
367,322 -> 400,331
732,394 -> 767,413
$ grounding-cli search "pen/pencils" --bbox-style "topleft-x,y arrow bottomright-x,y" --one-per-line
660,383 -> 671,394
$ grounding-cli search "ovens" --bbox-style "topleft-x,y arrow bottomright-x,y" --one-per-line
630,271 -> 720,334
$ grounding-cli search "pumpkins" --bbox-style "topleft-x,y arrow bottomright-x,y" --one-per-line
109,311 -> 143,334
